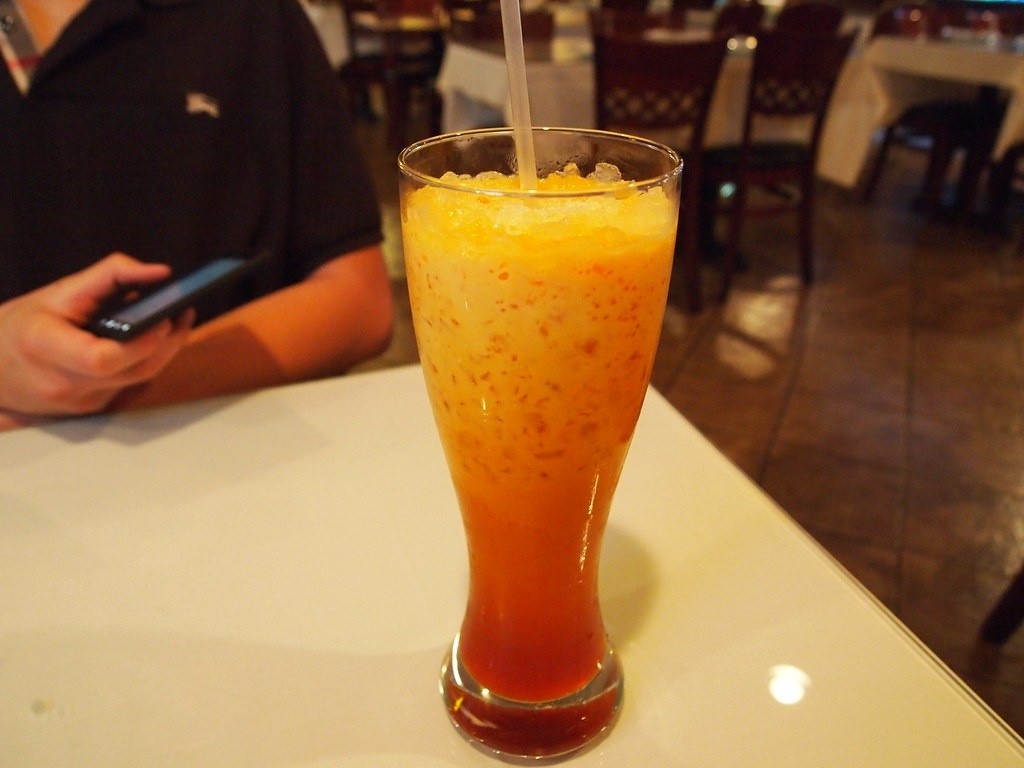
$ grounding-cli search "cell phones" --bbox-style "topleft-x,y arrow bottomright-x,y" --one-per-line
96,251 -> 264,342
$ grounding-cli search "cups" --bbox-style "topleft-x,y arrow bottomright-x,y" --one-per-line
395,128 -> 684,763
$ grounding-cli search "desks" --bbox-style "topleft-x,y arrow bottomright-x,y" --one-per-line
0,362 -> 1024,768
815,35 -> 1024,190
354,1 -> 888,116
437,29 -> 866,274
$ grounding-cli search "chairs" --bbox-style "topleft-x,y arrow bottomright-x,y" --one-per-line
865,84 -> 1010,223
338,0 -> 926,313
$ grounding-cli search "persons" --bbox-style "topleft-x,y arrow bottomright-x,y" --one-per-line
0,0 -> 396,429
